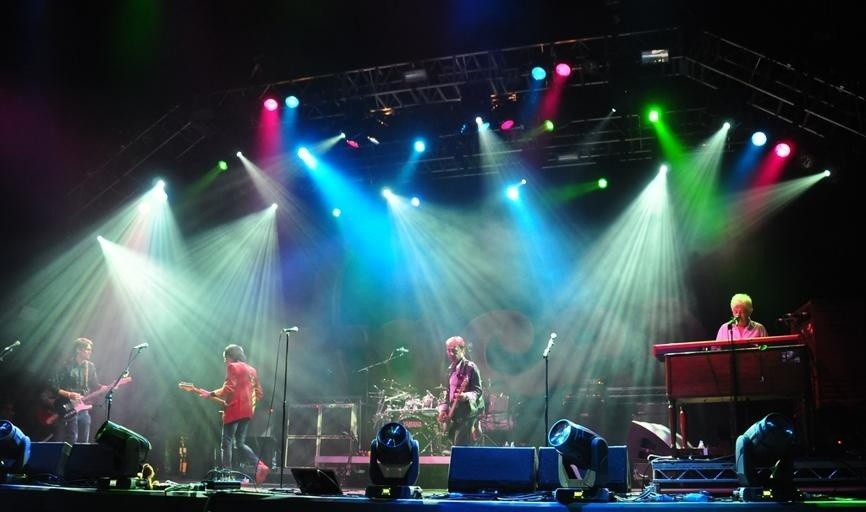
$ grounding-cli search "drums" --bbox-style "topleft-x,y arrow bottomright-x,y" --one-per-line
399,413 -> 425,449
422,410 -> 440,418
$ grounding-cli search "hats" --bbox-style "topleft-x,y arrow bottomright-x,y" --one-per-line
226,345 -> 246,361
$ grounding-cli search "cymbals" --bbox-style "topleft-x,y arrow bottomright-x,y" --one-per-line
433,385 -> 447,390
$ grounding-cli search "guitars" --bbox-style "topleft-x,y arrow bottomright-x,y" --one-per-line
56,376 -> 132,423
38,406 -> 57,425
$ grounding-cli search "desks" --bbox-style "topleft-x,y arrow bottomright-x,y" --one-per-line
662,344 -> 812,458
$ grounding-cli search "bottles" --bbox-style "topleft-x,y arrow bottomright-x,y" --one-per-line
505,441 -> 509,447
511,442 -> 515,447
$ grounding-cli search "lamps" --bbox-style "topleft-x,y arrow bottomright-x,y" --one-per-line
94,420 -> 152,490
549,418 -> 610,501
364,422 -> 420,499
0,420 -> 32,485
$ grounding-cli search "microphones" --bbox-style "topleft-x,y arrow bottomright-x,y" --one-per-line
733,315 -> 741,326
283,326 -> 300,333
396,347 -> 408,353
133,343 -> 149,350
5,341 -> 21,350
543,332 -> 557,357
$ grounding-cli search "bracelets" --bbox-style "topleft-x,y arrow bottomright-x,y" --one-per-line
209,392 -> 215,397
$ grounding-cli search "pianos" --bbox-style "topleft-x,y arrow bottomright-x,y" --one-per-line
653,334 -> 810,362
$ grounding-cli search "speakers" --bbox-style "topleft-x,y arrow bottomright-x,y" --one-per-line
65,442 -> 117,479
28,442 -> 71,479
539,446 -> 632,492
447,446 -> 537,492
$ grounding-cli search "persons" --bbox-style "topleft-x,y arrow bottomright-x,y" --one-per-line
39,385 -> 66,442
710,292 -> 770,351
436,335 -> 487,446
46,337 -> 111,448
197,344 -> 271,487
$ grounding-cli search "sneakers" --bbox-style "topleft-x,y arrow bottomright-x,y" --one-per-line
255,461 -> 270,486
